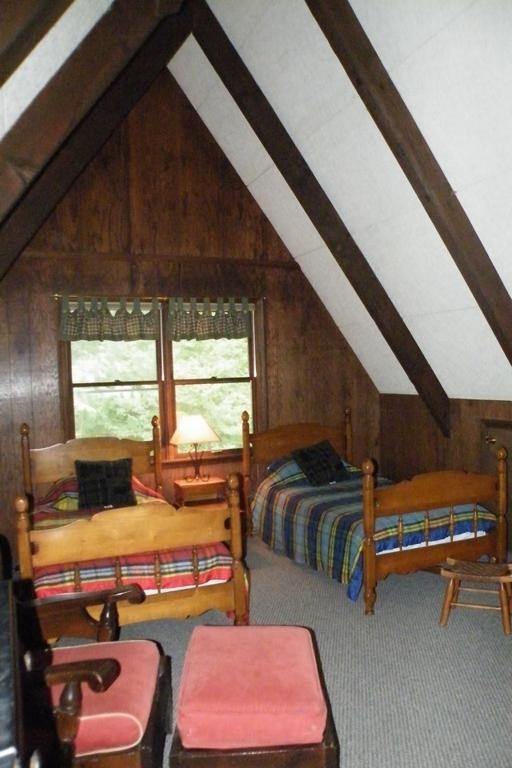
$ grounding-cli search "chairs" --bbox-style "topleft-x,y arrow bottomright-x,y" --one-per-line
0,533 -> 174,767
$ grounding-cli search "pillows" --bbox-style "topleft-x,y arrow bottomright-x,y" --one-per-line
74,458 -> 137,514
263,457 -> 292,476
290,438 -> 349,487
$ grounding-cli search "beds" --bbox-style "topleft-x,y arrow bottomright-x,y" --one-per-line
13,415 -> 252,646
241,406 -> 507,616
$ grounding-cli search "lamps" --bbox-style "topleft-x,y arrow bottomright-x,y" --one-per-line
169,411 -> 222,483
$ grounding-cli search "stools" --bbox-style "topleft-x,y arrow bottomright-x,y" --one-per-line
439,556 -> 512,636
167,624 -> 340,767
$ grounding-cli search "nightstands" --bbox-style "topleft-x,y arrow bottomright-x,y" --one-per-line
172,477 -> 228,508
